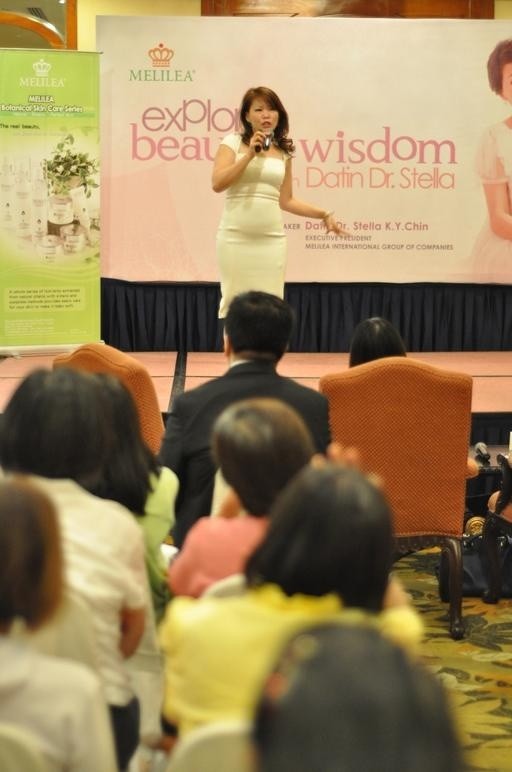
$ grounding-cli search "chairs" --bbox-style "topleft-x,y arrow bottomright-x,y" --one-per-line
167,722 -> 247,772
53,343 -> 166,452
466,441 -> 512,605
320,355 -> 476,639
1,726 -> 55,772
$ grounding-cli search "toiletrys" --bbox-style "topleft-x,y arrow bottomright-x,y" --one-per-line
2,195 -> 90,263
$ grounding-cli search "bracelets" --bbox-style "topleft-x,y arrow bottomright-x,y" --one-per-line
322,211 -> 331,219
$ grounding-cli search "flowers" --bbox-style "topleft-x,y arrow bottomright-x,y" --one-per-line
40,136 -> 99,200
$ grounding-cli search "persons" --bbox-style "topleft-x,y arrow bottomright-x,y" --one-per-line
0,290 -> 480,772
211,87 -> 350,363
474,37 -> 512,281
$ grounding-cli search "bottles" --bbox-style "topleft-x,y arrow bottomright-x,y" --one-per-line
47,195 -> 73,234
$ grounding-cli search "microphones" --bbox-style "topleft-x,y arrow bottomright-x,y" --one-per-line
264,128 -> 275,151
